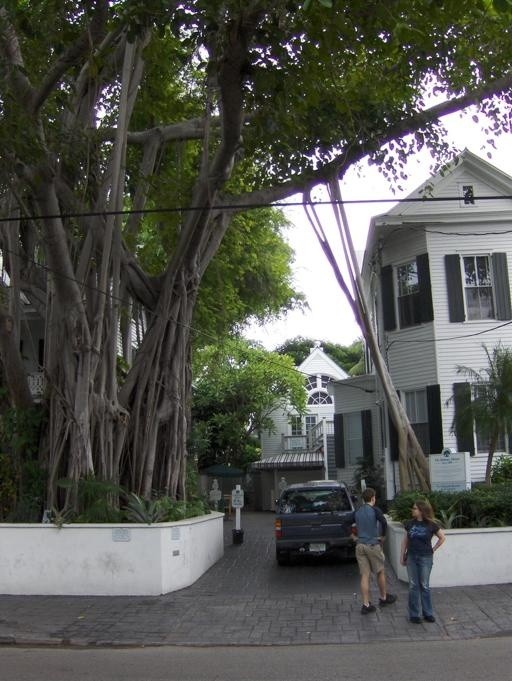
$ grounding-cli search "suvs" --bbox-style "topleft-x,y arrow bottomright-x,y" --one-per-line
273,479 -> 359,566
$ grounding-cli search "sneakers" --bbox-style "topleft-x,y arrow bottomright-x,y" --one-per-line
362,602 -> 376,614
379,593 -> 397,606
424,614 -> 435,622
410,616 -> 424,624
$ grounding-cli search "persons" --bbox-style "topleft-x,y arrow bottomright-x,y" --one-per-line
399,499 -> 445,622
341,488 -> 397,613
